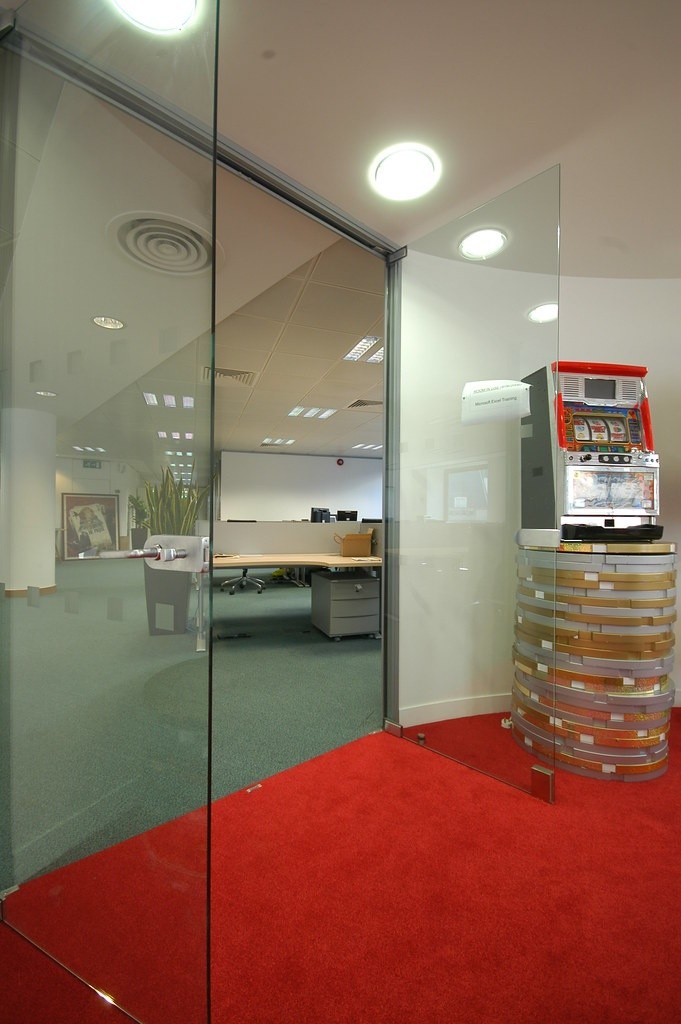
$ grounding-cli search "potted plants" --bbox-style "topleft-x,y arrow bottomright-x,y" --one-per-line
128,490 -> 148,549
142,458 -> 213,635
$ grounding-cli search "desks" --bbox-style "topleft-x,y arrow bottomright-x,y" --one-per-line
194,519 -> 383,652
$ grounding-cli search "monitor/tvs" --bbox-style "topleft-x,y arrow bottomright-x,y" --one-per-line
311,508 -> 330,523
337,511 -> 357,522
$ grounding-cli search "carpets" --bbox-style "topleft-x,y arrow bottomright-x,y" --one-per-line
0,708 -> 681,1024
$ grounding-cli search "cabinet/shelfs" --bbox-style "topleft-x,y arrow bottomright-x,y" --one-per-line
310,572 -> 379,638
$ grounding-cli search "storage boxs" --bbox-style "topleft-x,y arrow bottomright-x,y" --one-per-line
335,528 -> 374,558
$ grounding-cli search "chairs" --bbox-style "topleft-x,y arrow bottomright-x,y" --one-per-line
220,520 -> 266,595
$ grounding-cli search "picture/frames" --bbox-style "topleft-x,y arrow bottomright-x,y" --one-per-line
62,493 -> 121,560
